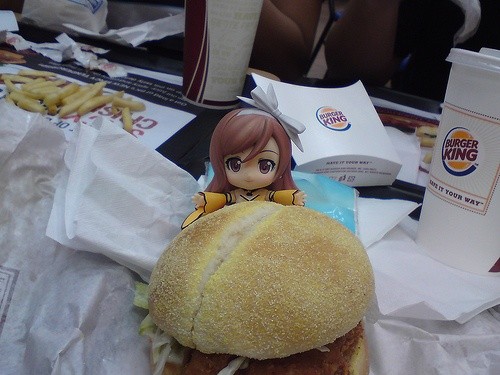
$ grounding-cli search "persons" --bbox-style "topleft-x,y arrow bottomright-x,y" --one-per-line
252,0 -> 500,103
181,84 -> 306,230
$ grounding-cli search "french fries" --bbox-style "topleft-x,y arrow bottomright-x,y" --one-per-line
0,70 -> 146,132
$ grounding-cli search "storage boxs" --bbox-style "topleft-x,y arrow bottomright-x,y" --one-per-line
250,70 -> 404,188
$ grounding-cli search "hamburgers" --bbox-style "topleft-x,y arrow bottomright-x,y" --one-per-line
132,202 -> 383,375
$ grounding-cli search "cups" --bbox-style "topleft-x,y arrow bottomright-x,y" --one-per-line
181,0 -> 265,112
416,47 -> 500,274
